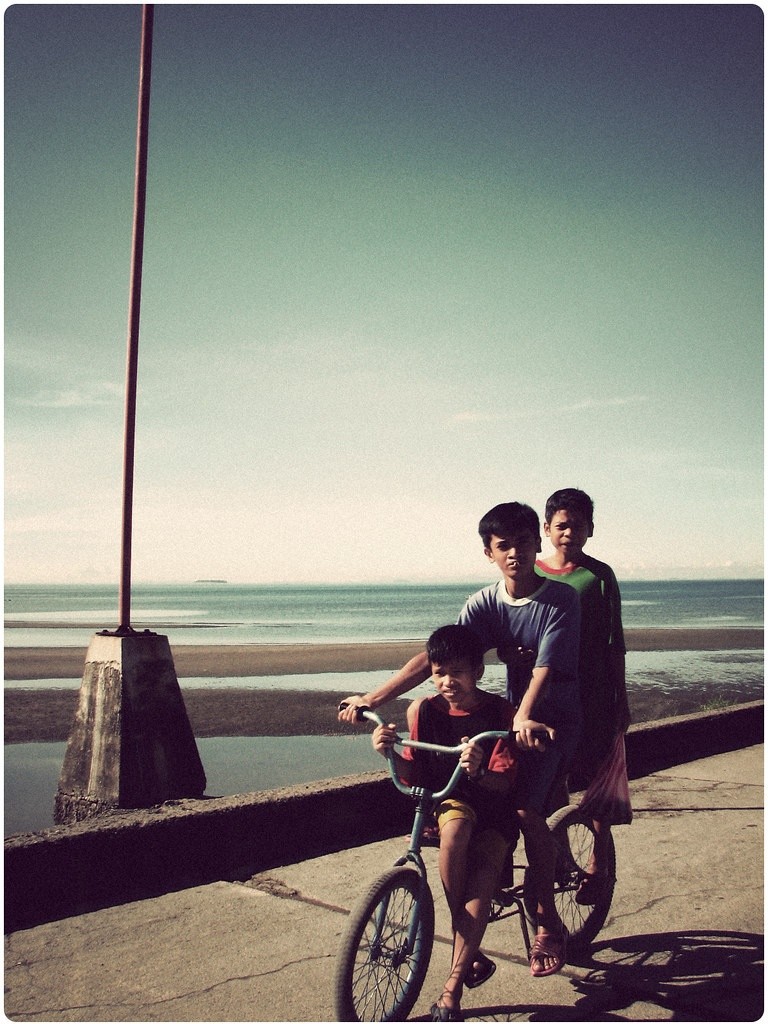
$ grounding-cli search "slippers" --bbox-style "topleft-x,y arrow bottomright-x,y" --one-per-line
529,923 -> 568,977
432,1002 -> 465,1022
463,950 -> 496,989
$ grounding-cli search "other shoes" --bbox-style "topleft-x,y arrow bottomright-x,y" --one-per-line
575,867 -> 611,906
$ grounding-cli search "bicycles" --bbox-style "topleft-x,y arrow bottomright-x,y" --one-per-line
333,701 -> 616,1022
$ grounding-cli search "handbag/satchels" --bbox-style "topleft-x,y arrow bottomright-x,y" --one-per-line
579,724 -> 633,825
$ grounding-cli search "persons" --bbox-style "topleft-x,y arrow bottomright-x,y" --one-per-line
337,503 -> 581,975
535,488 -> 632,904
372,626 -> 520,1022
